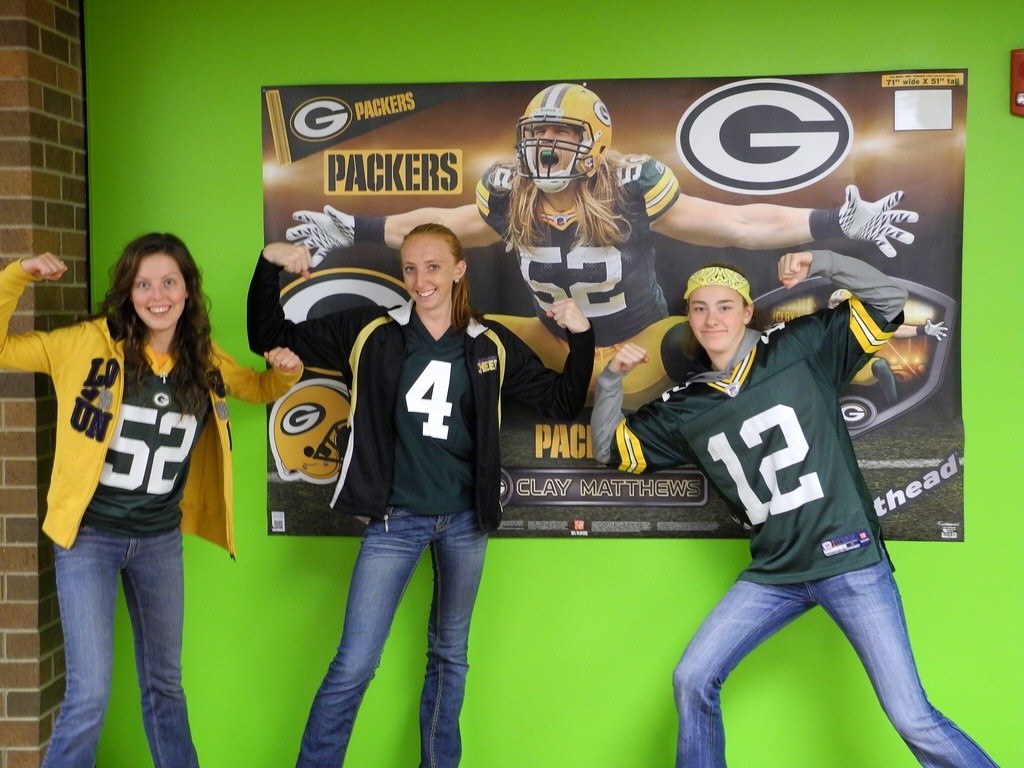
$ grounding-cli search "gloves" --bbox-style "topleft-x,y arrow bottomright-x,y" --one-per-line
286,204 -> 390,268
810,184 -> 919,258
916,319 -> 948,341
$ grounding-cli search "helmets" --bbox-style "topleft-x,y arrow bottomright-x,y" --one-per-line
828,289 -> 853,310
513,83 -> 612,193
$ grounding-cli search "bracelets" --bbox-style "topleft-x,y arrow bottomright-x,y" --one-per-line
809,205 -> 843,241
916,325 -> 927,336
353,215 -> 388,244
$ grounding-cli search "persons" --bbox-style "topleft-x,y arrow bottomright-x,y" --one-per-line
0,231 -> 308,768
246,223 -> 597,768
824,287 -> 948,408
592,248 -> 1024,768
283,80 -> 920,434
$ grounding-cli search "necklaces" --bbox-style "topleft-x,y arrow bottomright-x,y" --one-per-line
143,335 -> 179,384
